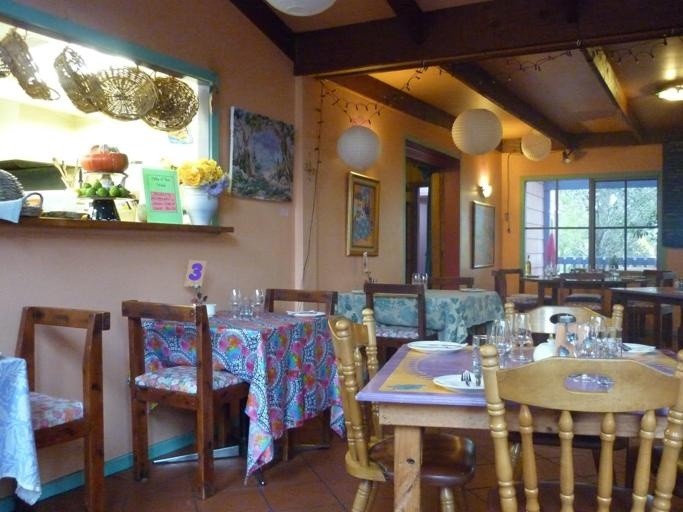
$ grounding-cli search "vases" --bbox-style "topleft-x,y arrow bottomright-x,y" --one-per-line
176,184 -> 221,225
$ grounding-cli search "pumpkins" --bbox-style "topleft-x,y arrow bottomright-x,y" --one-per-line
80,144 -> 130,173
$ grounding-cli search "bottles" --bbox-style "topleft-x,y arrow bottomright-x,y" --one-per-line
525,255 -> 532,275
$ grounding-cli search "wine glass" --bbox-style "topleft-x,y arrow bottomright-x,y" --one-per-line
230,287 -> 266,322
472,312 -> 624,369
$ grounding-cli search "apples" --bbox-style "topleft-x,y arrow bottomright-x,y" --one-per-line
74,179 -> 130,197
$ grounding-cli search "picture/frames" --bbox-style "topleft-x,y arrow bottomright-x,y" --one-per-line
346,170 -> 381,258
471,200 -> 496,270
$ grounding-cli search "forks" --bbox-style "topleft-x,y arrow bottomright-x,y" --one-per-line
459,371 -> 472,387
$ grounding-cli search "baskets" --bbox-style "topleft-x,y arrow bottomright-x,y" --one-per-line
93,66 -> 160,119
54,48 -> 107,112
0,27 -> 60,100
141,77 -> 199,132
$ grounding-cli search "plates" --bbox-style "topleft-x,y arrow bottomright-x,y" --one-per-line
620,342 -> 657,356
460,288 -> 487,293
288,311 -> 326,318
432,373 -> 486,390
408,339 -> 467,354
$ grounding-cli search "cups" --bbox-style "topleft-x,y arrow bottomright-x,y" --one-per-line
206,304 -> 216,318
412,272 -> 429,292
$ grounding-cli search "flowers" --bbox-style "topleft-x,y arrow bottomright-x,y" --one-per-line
176,158 -> 229,188
